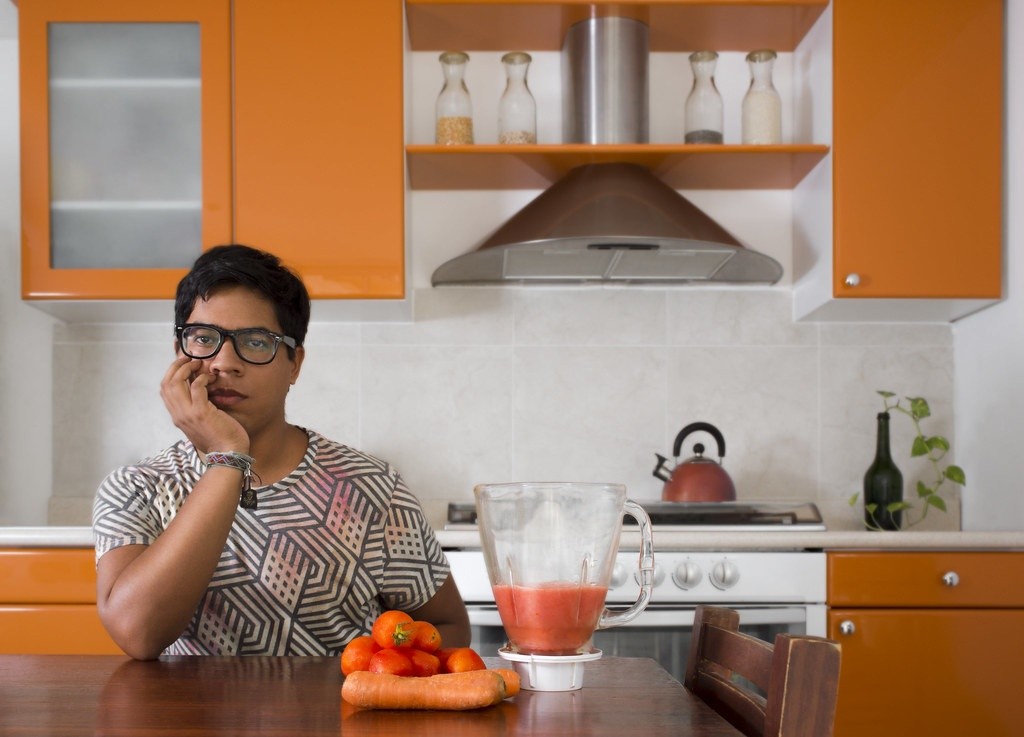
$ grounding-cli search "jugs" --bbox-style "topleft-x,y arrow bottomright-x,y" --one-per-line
476,479 -> 653,690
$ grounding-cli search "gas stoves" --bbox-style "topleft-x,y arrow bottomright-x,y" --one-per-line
448,493 -> 819,527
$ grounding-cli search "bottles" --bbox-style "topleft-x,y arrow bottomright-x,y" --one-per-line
739,48 -> 783,146
683,50 -> 724,145
494,53 -> 537,145
435,50 -> 476,148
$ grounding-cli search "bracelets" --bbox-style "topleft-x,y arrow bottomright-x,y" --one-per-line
202,452 -> 258,509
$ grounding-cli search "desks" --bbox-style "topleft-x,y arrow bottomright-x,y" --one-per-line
0,653 -> 744,737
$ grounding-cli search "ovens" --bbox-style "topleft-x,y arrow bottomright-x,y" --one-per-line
436,547 -> 829,699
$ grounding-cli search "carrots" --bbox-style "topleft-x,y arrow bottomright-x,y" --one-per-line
341,669 -> 522,710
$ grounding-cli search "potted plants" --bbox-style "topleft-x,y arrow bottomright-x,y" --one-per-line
851,390 -> 967,532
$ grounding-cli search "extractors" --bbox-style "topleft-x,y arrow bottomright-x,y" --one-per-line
428,15 -> 785,290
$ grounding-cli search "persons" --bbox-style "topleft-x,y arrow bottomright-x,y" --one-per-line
92,244 -> 471,660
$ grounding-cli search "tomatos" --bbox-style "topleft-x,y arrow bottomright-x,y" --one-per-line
341,611 -> 487,678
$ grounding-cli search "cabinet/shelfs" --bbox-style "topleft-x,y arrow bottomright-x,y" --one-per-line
824,548 -> 1024,737
12,0 -> 415,326
791,0 -> 1003,323
0,547 -> 126,656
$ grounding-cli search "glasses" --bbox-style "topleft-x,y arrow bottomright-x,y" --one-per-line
177,323 -> 296,366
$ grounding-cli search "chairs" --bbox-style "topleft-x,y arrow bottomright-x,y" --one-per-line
683,604 -> 842,737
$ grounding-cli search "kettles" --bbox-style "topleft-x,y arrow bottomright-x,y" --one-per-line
655,421 -> 737,504
